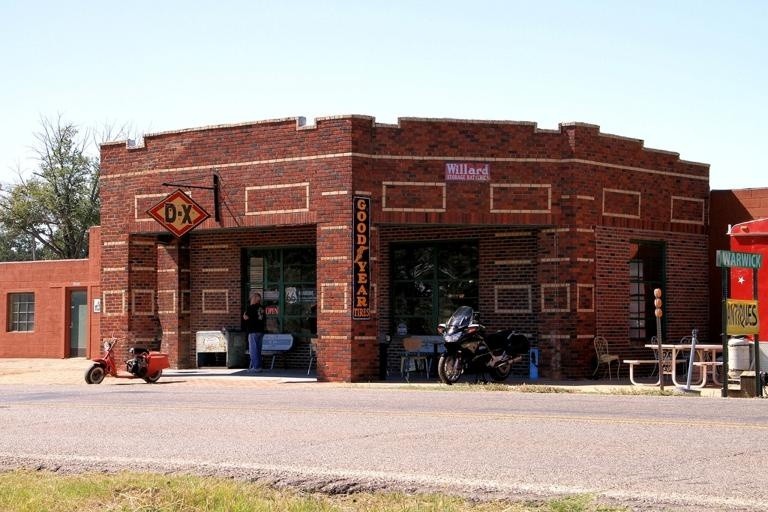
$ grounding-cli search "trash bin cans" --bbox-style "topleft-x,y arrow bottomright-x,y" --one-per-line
728,338 -> 751,378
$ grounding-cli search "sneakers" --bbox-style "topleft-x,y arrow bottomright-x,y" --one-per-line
240,368 -> 263,375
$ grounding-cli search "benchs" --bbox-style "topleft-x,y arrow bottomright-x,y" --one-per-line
244,349 -> 280,369
622,359 -> 723,388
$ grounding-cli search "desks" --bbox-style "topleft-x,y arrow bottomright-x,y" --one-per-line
644,344 -> 723,387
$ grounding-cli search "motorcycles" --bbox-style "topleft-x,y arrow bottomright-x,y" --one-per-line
437,305 -> 531,386
85,330 -> 171,384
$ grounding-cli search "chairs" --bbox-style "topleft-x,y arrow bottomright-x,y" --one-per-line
306,337 -> 317,376
592,335 -> 621,380
401,337 -> 428,381
649,335 -> 700,377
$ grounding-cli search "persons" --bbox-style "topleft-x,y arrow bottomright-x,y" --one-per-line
243,293 -> 265,373
